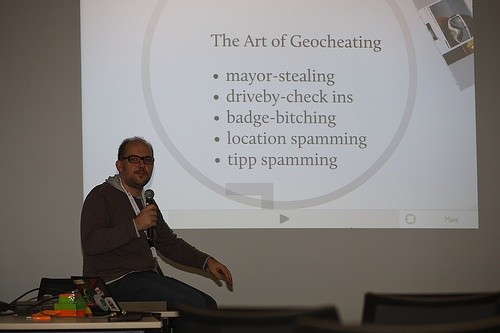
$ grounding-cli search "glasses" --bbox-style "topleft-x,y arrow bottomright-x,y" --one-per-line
122,155 -> 154,164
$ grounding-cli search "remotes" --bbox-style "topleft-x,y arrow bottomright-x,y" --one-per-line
109,315 -> 143,322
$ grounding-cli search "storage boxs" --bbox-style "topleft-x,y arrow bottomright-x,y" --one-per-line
54,294 -> 85,316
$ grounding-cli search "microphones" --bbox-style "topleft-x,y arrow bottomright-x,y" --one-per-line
144,189 -> 155,237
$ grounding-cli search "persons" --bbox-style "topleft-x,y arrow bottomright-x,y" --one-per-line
80,137 -> 233,311
436,14 -> 474,47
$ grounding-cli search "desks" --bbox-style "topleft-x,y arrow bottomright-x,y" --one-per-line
0,301 -> 178,333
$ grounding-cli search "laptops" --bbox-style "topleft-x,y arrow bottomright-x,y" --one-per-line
71,276 -> 162,317
